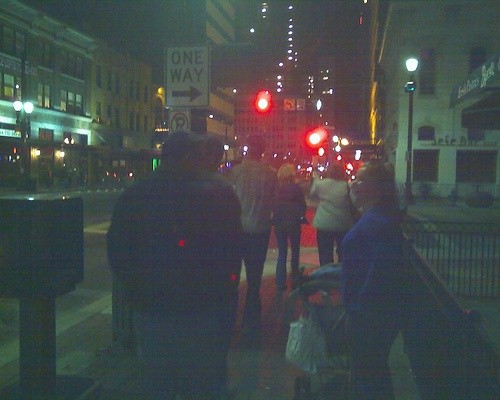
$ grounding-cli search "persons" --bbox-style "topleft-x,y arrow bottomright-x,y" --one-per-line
107,130 -> 242,400
225,135 -> 281,318
272,159 -> 407,400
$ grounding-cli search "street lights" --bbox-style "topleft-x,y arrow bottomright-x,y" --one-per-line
223,144 -> 231,165
405,58 -> 419,188
14,99 -> 34,196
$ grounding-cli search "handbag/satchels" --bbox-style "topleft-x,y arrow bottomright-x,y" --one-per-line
326,308 -> 362,359
284,309 -> 315,375
